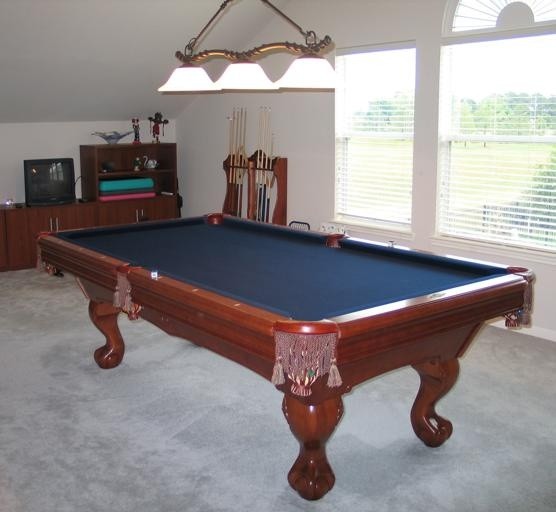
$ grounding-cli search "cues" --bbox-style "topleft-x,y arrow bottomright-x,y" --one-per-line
255,106 -> 273,224
228,107 -> 246,219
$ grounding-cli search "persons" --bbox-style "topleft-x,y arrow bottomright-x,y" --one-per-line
133,154 -> 142,172
132,117 -> 142,145
148,112 -> 168,143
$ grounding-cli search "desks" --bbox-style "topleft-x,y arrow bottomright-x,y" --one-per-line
35,209 -> 536,503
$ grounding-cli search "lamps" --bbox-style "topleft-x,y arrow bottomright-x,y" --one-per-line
153,0 -> 351,95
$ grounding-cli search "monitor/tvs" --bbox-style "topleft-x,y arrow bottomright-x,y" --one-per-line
22,157 -> 77,206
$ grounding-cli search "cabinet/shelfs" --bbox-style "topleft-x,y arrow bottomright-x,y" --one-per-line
79,141 -> 179,201
0,209 -> 8,273
98,196 -> 178,227
4,202 -> 97,272
